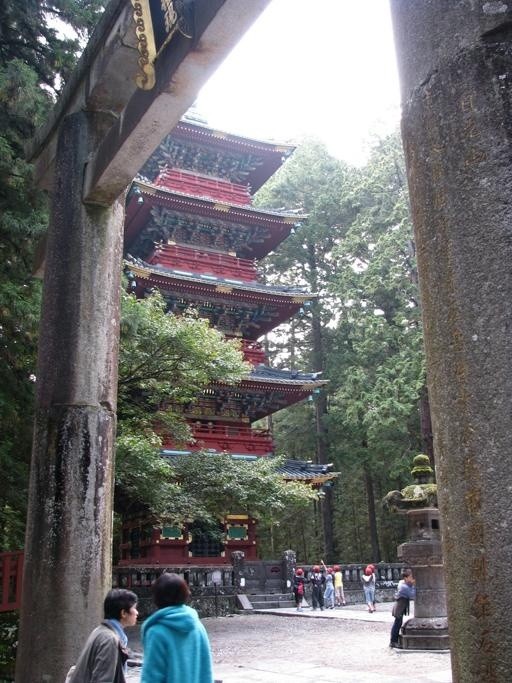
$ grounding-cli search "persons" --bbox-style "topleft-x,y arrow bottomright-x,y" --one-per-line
367,565 -> 376,611
360,567 -> 374,613
69,589 -> 139,683
293,559 -> 346,612
389,569 -> 416,648
139,573 -> 214,683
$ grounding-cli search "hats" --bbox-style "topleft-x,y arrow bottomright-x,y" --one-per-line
365,564 -> 374,575
296,569 -> 302,574
311,565 -> 338,573
